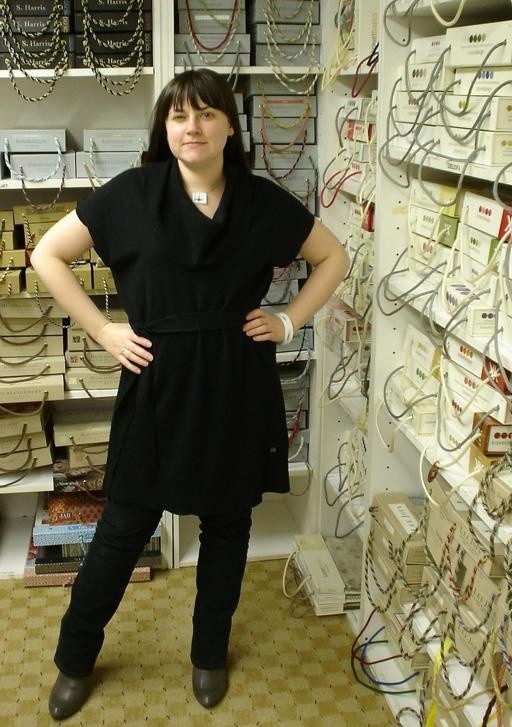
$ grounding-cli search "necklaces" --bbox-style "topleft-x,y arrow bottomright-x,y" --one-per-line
185,175 -> 225,204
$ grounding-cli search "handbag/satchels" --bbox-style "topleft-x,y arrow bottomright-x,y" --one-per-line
0,127 -> 150,488
176,2 -> 321,218
0,0 -> 149,101
297,535 -> 360,616
364,1 -> 512,724
261,252 -> 313,498
324,0 -> 379,540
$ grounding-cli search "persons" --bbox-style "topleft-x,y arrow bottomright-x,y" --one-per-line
28,71 -> 352,721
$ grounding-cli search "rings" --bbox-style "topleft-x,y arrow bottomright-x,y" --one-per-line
122,349 -> 130,356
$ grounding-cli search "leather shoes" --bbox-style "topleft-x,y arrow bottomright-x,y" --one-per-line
48,671 -> 95,720
190,665 -> 228,709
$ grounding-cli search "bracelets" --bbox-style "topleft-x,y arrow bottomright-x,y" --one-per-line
276,311 -> 294,347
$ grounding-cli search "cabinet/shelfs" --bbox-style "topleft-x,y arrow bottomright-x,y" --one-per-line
318,0 -> 511,727
2,2 -> 319,579
